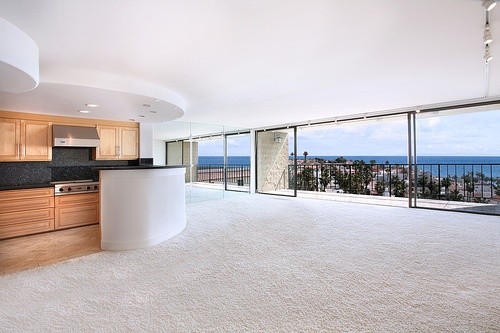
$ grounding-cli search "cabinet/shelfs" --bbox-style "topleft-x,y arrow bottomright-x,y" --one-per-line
0,117 -> 53,162
0,187 -> 54,239
92,125 -> 139,162
56,193 -> 99,231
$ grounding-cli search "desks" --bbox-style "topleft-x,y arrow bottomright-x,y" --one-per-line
91,164 -> 191,251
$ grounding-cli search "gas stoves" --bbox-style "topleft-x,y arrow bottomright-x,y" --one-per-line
50,179 -> 100,196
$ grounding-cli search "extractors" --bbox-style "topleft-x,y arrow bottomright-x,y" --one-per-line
52,127 -> 100,147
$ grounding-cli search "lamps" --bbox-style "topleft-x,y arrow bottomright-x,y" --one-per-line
482,0 -> 496,11
483,44 -> 493,62
482,11 -> 494,44
